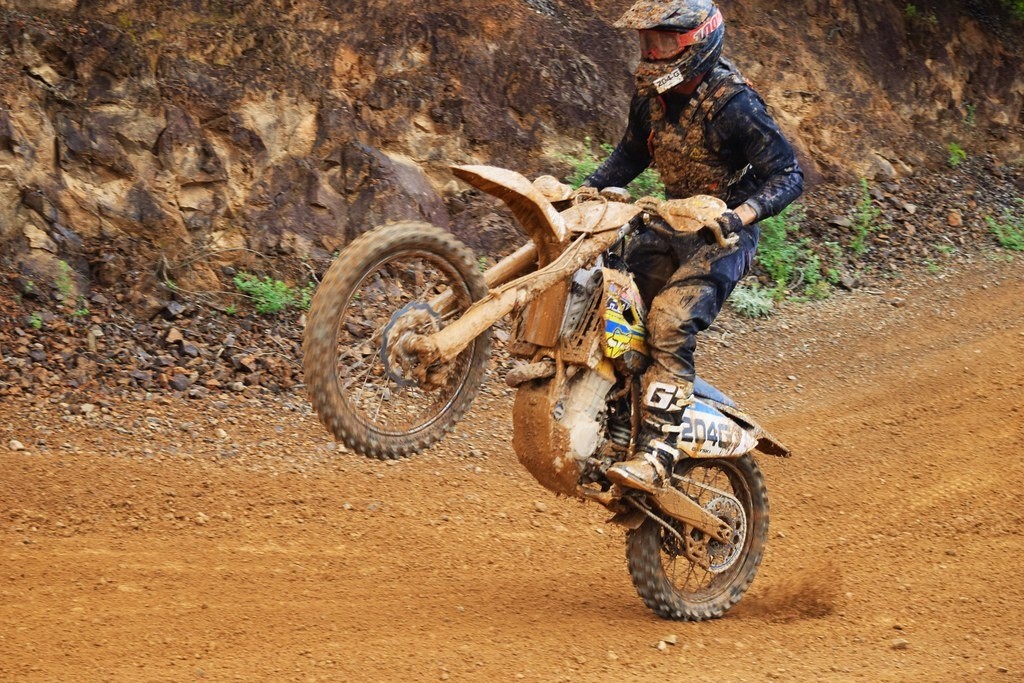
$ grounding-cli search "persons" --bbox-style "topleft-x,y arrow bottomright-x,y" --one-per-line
570,0 -> 803,490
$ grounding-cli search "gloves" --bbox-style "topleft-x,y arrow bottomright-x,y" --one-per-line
698,209 -> 741,244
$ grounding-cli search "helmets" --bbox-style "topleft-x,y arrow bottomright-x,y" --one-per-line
612,0 -> 725,95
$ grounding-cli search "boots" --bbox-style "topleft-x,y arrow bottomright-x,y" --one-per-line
608,377 -> 693,493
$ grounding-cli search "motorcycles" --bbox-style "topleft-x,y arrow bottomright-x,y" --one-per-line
298,165 -> 791,624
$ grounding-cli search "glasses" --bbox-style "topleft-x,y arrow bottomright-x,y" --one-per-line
639,9 -> 722,61
